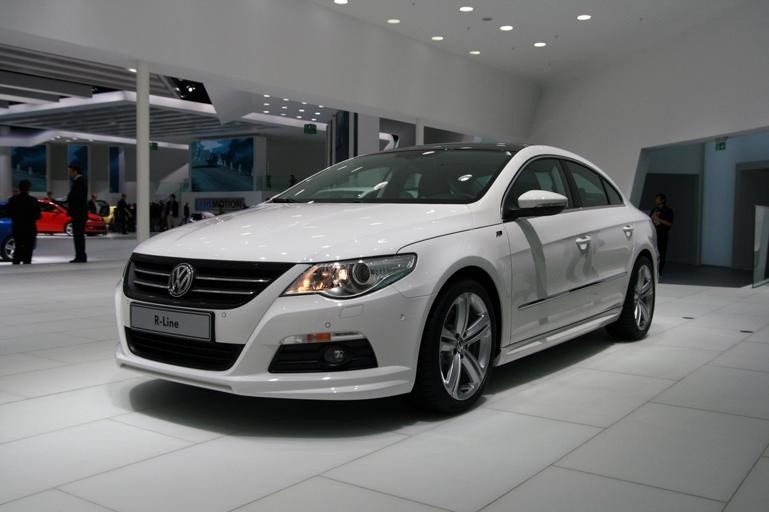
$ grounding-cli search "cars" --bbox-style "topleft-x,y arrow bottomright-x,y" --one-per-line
95,199 -> 117,230
0,200 -> 37,261
34,198 -> 107,236
114,142 -> 662,419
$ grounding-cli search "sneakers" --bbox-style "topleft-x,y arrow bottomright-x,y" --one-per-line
70,257 -> 87,262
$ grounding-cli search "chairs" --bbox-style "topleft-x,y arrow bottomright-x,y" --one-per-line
411,166 -> 540,218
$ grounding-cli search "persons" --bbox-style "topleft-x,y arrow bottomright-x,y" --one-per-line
66,161 -> 89,264
115,190 -> 180,236
43,190 -> 57,236
184,201 -> 192,224
7,178 -> 41,265
647,190 -> 674,279
88,192 -> 101,216
